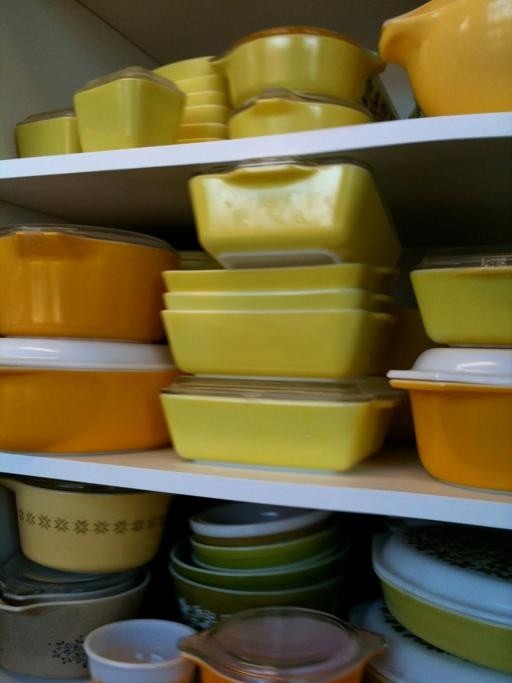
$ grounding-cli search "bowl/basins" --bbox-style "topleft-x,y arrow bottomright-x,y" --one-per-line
4,153 -> 511,511
0,0 -> 512,149
0,463 -> 512,683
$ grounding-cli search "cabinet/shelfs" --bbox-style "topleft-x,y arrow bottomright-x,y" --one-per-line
1,112 -> 512,533
